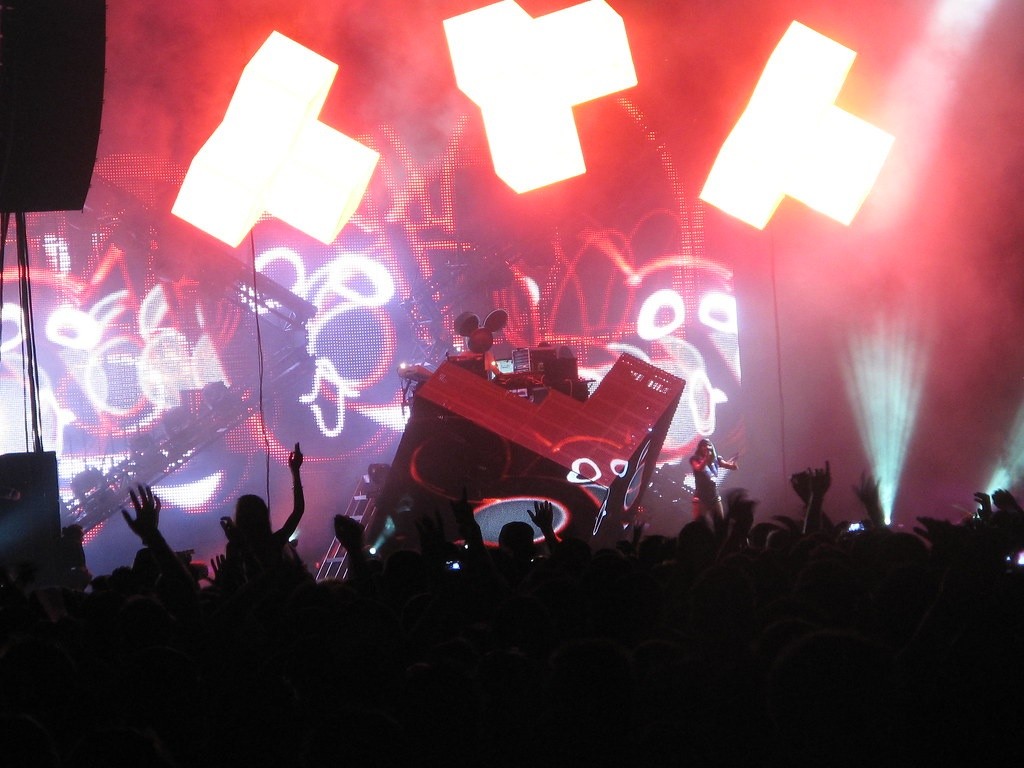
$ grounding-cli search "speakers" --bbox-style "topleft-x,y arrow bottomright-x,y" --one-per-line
0,0 -> 107,214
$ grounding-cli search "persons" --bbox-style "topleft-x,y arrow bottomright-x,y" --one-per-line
1,461 -> 1023,764
225,441 -> 305,559
690,438 -> 739,525
